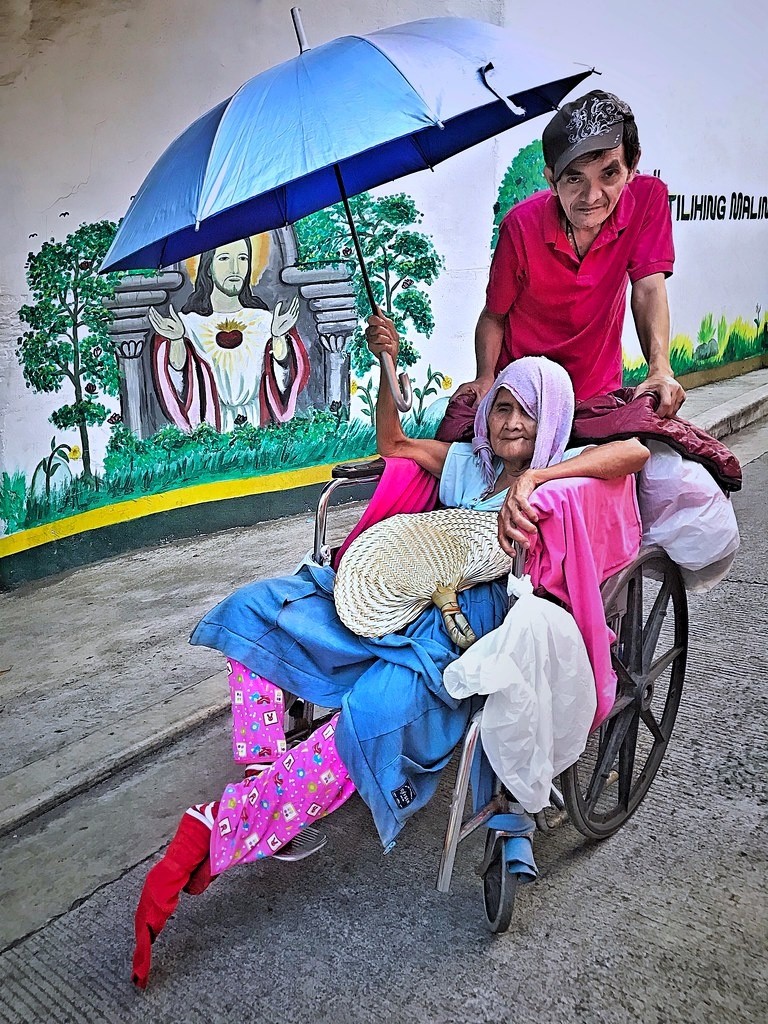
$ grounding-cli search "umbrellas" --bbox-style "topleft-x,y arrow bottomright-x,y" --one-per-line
96,6 -> 604,411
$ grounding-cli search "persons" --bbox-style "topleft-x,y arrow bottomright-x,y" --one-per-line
474,89 -> 687,419
129,307 -> 652,990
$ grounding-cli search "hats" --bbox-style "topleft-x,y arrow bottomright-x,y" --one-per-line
542,89 -> 633,181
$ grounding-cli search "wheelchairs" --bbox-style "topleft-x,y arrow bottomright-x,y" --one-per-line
240,453 -> 690,933
